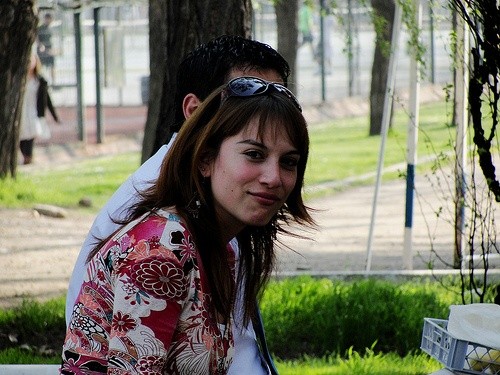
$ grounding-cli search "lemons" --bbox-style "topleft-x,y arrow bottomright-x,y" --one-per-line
469,349 -> 500,374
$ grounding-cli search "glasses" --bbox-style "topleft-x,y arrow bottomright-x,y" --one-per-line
218,77 -> 303,119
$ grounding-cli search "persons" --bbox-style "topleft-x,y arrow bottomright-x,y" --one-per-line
65,34 -> 290,327
36,14 -> 57,87
59,79 -> 310,375
18,51 -> 62,165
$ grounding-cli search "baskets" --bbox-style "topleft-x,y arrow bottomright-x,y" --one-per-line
419,317 -> 500,374
